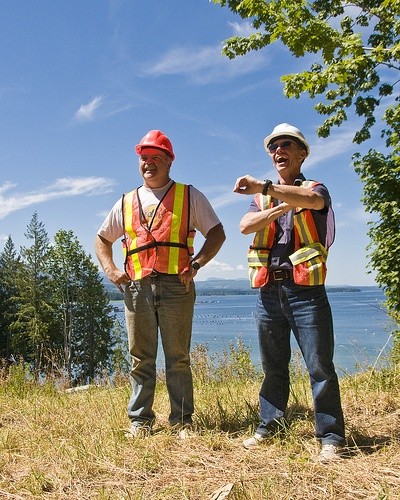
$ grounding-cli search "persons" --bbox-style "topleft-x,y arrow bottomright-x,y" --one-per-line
232,122 -> 347,464
95,130 -> 226,439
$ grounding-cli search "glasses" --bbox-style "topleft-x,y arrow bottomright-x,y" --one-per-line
268,141 -> 295,151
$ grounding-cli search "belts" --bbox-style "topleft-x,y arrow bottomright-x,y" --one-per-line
145,270 -> 160,278
269,270 -> 293,281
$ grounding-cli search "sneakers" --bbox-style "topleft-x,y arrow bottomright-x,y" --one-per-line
318,444 -> 342,464
242,433 -> 264,449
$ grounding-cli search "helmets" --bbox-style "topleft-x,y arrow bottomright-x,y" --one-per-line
136,130 -> 174,160
264,122 -> 310,159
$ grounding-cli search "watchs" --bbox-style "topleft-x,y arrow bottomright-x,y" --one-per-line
189,260 -> 200,270
261,178 -> 273,196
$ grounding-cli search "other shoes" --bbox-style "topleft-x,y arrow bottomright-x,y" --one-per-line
179,427 -> 195,440
124,422 -> 143,438
